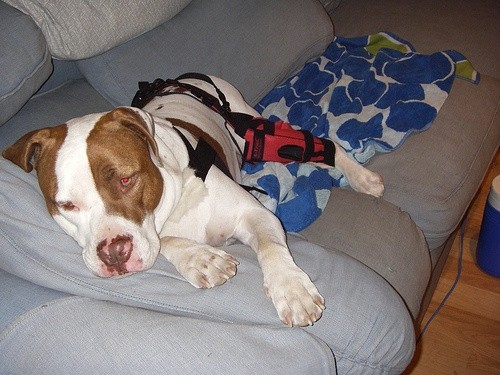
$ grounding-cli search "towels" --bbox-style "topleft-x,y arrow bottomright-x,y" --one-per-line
204,28 -> 484,230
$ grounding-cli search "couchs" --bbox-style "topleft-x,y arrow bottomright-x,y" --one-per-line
0,0 -> 500,375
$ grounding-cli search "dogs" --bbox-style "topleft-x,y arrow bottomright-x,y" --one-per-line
2,75 -> 385,328
